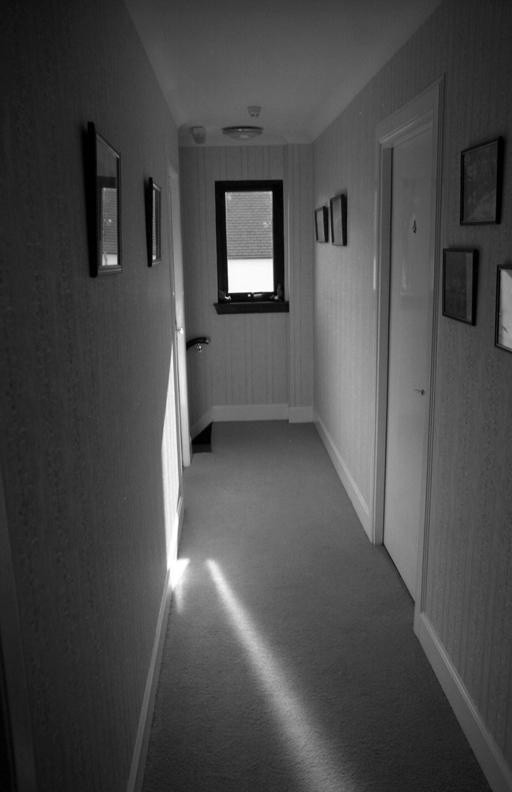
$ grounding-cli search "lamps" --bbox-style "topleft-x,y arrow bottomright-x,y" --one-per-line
223,126 -> 262,144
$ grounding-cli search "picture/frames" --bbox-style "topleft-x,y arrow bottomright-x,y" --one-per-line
315,193 -> 347,246
83,121 -> 121,278
442,135 -> 512,354
147,177 -> 162,266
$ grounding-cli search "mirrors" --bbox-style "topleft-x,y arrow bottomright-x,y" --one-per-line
214,179 -> 284,302
